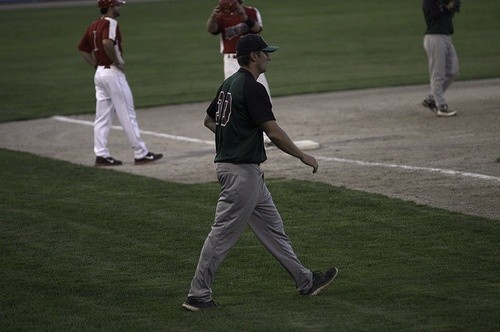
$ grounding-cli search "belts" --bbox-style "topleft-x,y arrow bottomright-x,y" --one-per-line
95,64 -> 110,69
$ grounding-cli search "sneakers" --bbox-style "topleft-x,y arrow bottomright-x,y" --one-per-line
182,297 -> 223,312
304,267 -> 339,296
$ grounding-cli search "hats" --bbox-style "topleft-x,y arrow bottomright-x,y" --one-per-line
233,33 -> 279,58
97,0 -> 126,9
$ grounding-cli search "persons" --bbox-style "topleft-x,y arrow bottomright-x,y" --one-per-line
182,33 -> 339,311
206,0 -> 272,147
422,0 -> 463,116
77,0 -> 163,166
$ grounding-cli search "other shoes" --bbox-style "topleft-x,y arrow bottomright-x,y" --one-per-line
95,156 -> 122,166
422,98 -> 438,113
135,152 -> 163,165
436,106 -> 457,118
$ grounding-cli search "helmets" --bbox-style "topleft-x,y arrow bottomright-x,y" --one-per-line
219,0 -> 240,15
